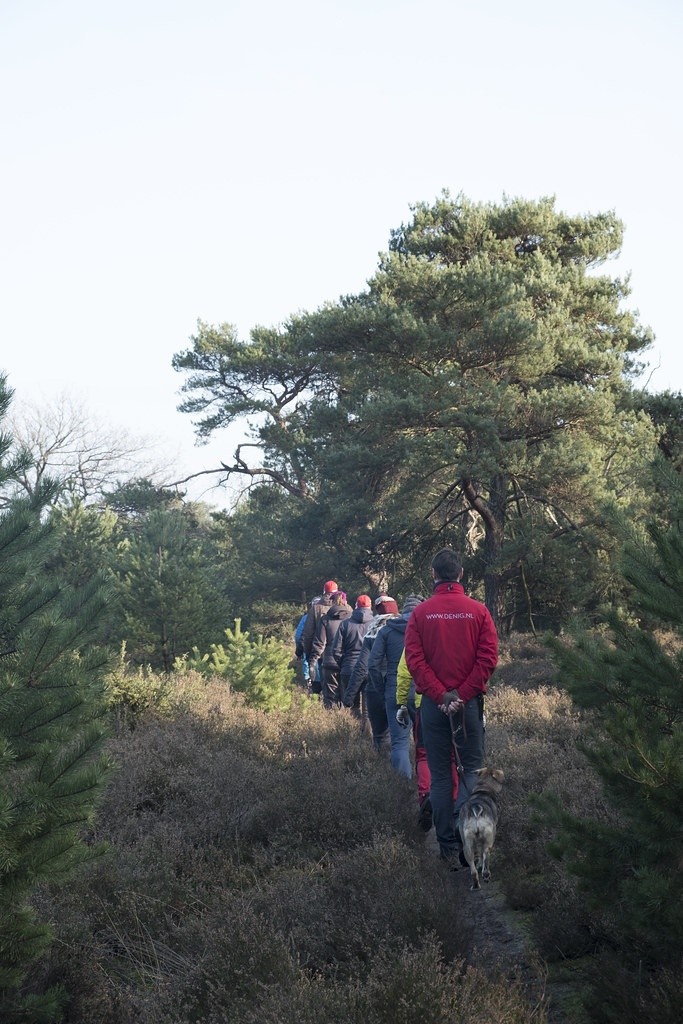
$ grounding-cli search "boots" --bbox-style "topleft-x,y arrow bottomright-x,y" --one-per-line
438,844 -> 478,870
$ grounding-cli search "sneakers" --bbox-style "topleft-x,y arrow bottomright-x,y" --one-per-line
417,793 -> 433,831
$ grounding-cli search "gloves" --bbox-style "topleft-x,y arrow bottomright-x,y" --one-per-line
396,704 -> 409,729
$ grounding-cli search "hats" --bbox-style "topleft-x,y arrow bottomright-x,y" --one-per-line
324,581 -> 338,592
311,597 -> 321,604
402,595 -> 425,613
375,593 -> 398,615
357,595 -> 371,607
329,591 -> 346,599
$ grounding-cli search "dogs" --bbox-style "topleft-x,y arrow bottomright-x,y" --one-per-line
458,767 -> 506,891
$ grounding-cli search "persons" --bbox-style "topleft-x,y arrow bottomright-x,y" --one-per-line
290,549 -> 500,873
